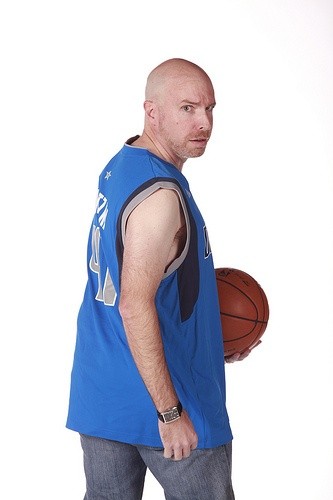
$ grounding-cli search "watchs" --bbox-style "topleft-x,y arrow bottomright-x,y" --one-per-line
157,401 -> 182,424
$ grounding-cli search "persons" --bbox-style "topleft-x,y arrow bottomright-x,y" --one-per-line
66,58 -> 262,500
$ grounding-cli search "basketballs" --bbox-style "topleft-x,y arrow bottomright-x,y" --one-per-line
212,267 -> 270,358
